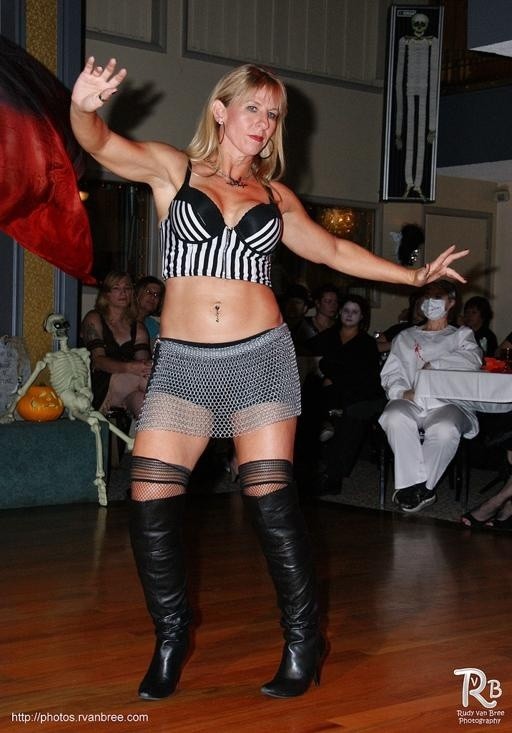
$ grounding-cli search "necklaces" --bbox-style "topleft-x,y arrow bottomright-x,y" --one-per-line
219,167 -> 249,188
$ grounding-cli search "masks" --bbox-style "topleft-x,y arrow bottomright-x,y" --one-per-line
421,298 -> 451,320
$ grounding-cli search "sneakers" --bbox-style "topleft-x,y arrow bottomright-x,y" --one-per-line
392,482 -> 436,512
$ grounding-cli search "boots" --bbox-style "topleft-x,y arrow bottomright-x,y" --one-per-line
242,481 -> 328,698
129,493 -> 192,700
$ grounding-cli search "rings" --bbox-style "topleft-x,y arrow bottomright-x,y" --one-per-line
99,93 -> 108,102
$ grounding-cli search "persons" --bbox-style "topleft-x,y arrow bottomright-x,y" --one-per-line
80,267 -> 511,528
70,52 -> 470,699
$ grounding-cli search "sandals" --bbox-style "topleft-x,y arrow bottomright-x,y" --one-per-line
462,512 -> 512,528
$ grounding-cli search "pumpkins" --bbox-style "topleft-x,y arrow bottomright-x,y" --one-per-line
16,381 -> 63,421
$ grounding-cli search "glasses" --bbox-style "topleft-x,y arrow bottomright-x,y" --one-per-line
146,290 -> 158,297
